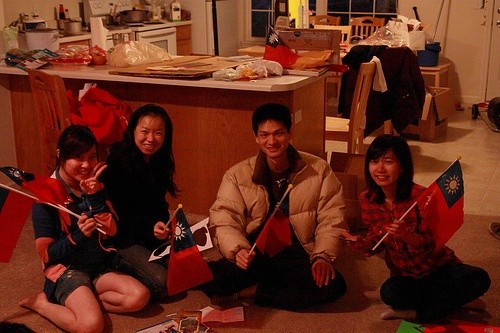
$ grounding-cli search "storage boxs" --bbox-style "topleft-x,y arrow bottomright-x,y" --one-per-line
278,26 -> 342,64
168,0 -> 181,21
387,17 -> 425,52
402,87 -> 457,142
329,152 -> 365,221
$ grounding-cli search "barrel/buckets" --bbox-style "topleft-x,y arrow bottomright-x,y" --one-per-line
417,43 -> 441,66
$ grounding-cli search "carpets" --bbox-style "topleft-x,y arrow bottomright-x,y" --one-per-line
0,182 -> 500,333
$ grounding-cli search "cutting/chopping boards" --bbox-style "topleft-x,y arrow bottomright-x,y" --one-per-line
109,55 -> 250,79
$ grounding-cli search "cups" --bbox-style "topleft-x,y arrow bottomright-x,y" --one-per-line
472,104 -> 478,119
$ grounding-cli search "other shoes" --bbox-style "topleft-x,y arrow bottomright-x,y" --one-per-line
489,222 -> 500,240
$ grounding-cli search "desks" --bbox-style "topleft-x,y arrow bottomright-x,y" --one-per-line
237,45 -> 352,55
419,62 -> 452,87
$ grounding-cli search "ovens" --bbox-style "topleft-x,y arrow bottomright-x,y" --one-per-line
135,26 -> 177,56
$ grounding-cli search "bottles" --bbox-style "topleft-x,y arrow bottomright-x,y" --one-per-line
65,9 -> 70,21
59,3 -> 65,30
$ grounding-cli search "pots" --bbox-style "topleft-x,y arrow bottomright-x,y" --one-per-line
118,7 -> 150,23
63,20 -> 82,34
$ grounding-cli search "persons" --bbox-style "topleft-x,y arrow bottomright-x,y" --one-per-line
193,103 -> 354,308
97,104 -> 187,302
348,134 -> 491,322
18,124 -> 150,333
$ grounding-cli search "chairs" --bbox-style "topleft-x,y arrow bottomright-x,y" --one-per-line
314,15 -> 349,42
324,62 -> 375,153
26,67 -> 69,178
350,16 -> 385,38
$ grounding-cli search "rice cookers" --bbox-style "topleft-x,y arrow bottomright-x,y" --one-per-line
25,28 -> 64,51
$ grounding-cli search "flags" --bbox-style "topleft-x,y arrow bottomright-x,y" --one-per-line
167,209 -> 213,296
0,166 -> 71,263
415,160 -> 464,255
264,26 -> 302,67
255,205 -> 292,257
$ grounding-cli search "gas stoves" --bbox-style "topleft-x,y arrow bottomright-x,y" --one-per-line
83,0 -> 176,32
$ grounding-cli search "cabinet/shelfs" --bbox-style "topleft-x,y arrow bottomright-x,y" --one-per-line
175,24 -> 193,56
